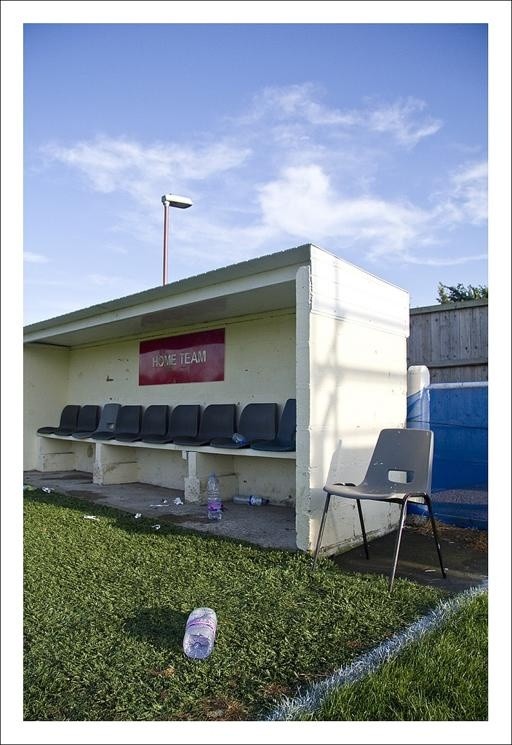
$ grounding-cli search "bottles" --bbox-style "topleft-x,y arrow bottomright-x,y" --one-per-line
206,470 -> 223,522
182,606 -> 220,659
232,495 -> 270,508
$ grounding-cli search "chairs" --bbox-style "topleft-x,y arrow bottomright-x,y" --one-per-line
36,399 -> 298,452
312,429 -> 447,592
